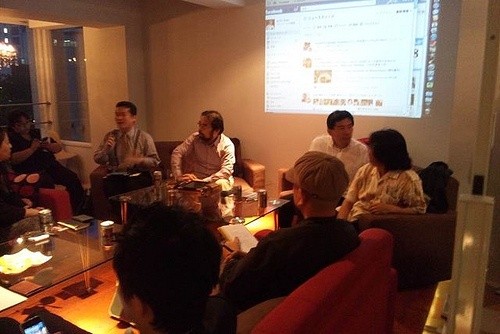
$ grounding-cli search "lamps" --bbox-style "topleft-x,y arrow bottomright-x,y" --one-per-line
0,40 -> 19,69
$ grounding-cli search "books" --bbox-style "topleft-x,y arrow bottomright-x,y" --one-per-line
176,180 -> 210,190
216,222 -> 259,254
57,218 -> 90,231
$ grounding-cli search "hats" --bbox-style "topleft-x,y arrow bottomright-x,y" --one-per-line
285,151 -> 349,199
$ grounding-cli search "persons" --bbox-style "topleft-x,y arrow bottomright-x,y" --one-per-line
209,150 -> 362,315
0,127 -> 53,243
7,109 -> 90,216
336,128 -> 427,221
111,200 -> 236,334
308,110 -> 369,219
171,111 -> 236,192
93,101 -> 162,213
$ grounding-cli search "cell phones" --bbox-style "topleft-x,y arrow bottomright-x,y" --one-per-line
20,315 -> 50,334
40,137 -> 48,144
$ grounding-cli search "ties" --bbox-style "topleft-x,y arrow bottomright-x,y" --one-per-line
122,134 -> 130,163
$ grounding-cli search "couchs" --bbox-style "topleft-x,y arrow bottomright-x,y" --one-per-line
250,229 -> 399,334
8,169 -> 73,221
278,166 -> 460,289
90,138 -> 266,222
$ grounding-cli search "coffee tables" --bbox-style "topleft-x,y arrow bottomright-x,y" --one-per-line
108,181 -> 290,228
0,218 -> 125,313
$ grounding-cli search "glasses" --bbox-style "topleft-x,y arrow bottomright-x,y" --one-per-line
197,122 -> 214,129
15,121 -> 31,128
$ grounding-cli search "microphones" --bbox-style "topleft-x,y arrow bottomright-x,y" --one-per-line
107,129 -> 120,149
